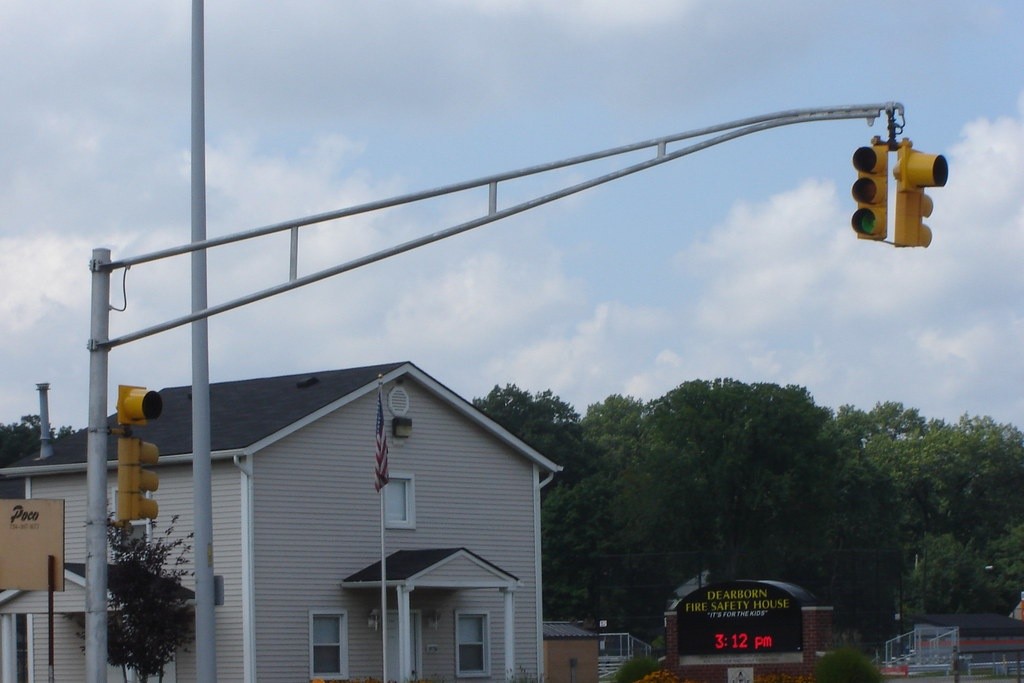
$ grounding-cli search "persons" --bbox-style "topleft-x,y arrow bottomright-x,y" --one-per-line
951,649 -> 959,682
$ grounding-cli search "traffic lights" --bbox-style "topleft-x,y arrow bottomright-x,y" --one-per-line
118,385 -> 164,426
117,436 -> 160,521
896,141 -> 949,249
851,143 -> 889,241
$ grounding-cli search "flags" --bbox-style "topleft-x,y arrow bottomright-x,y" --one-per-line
374,390 -> 389,492
